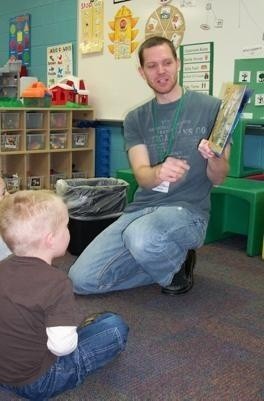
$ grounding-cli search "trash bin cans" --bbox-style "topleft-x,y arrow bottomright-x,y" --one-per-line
58,177 -> 129,256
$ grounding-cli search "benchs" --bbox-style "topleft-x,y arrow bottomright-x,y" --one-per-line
116,165 -> 264,258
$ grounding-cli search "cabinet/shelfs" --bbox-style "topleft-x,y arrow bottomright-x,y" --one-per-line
0,101 -> 95,210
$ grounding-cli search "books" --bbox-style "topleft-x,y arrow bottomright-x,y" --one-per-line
207,82 -> 255,158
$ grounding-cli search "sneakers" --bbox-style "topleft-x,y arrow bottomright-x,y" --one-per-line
76,311 -> 111,330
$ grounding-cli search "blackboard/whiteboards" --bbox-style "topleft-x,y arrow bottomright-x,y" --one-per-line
77,0 -> 264,135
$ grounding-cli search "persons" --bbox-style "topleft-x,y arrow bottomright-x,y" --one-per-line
0,177 -> 12,263
0,186 -> 132,401
66,34 -> 233,298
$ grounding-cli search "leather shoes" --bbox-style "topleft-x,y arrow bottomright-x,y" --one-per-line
161,249 -> 196,294
